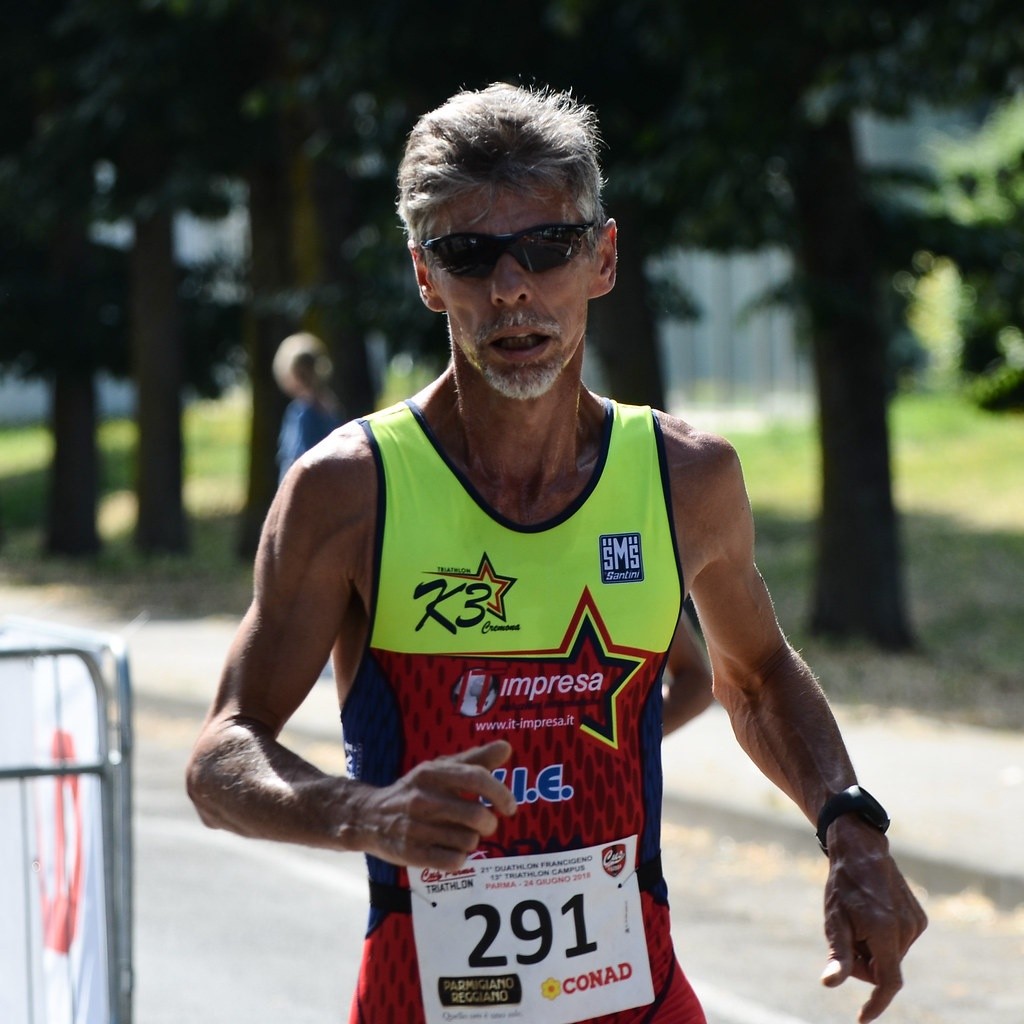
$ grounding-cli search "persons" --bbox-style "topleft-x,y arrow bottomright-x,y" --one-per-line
274,329 -> 347,491
187,81 -> 931,1023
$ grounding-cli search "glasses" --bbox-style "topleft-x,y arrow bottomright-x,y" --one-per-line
420,222 -> 595,279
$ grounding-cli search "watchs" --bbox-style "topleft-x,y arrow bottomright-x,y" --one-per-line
813,785 -> 891,858
